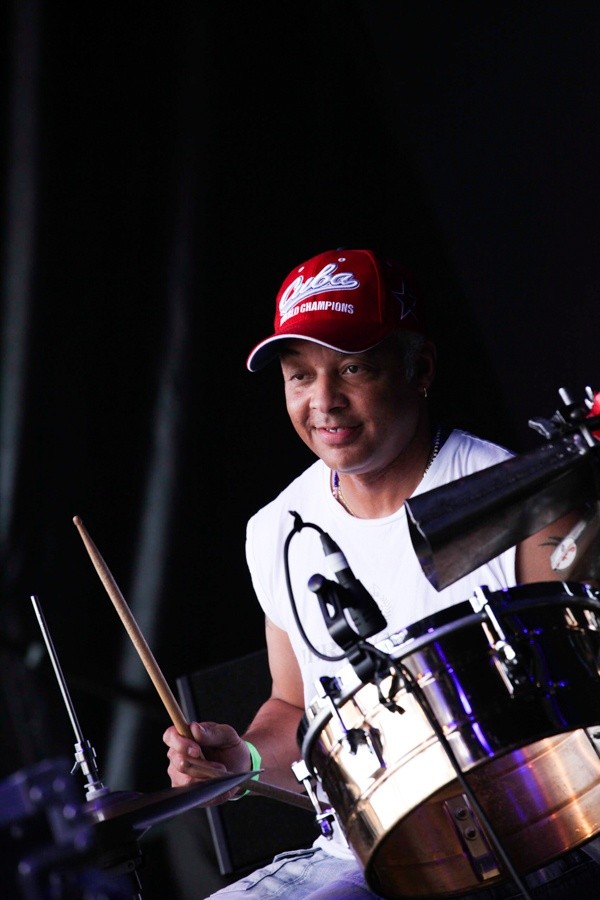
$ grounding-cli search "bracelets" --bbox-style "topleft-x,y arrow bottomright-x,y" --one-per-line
226,740 -> 262,803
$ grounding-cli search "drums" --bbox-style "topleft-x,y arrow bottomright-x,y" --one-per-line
295,578 -> 600,900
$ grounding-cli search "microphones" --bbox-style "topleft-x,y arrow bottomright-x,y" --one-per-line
320,532 -> 387,639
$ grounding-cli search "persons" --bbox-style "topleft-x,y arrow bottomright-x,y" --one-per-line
159,249 -> 587,899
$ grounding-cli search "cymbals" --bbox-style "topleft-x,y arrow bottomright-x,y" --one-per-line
21,769 -> 266,848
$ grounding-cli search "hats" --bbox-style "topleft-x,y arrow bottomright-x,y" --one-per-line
245,247 -> 431,373
